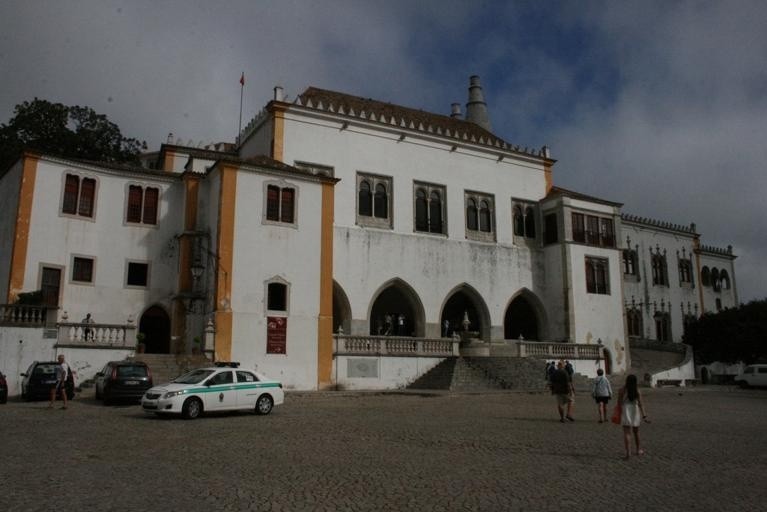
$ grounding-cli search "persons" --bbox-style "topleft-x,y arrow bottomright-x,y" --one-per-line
551,361 -> 575,422
590,368 -> 614,423
544,363 -> 551,382
81,313 -> 95,342
442,319 -> 450,338
383,311 -> 407,336
565,359 -> 574,382
617,374 -> 652,460
48,354 -> 68,408
544,361 -> 557,391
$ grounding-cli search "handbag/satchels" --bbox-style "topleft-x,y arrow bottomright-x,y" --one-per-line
611,386 -> 624,424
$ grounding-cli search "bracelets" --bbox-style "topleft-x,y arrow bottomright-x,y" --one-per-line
643,415 -> 647,418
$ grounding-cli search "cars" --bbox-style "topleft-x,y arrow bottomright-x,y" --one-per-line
141,360 -> 285,419
96,361 -> 155,402
1,371 -> 9,405
20,360 -> 75,402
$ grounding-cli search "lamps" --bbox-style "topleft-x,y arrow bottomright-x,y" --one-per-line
191,258 -> 207,301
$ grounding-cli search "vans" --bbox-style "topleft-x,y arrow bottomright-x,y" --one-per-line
734,364 -> 766,390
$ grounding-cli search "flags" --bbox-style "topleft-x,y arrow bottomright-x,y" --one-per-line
238,74 -> 245,86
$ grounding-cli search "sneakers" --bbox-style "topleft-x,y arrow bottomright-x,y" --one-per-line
559,415 -> 575,423
597,417 -> 609,423
